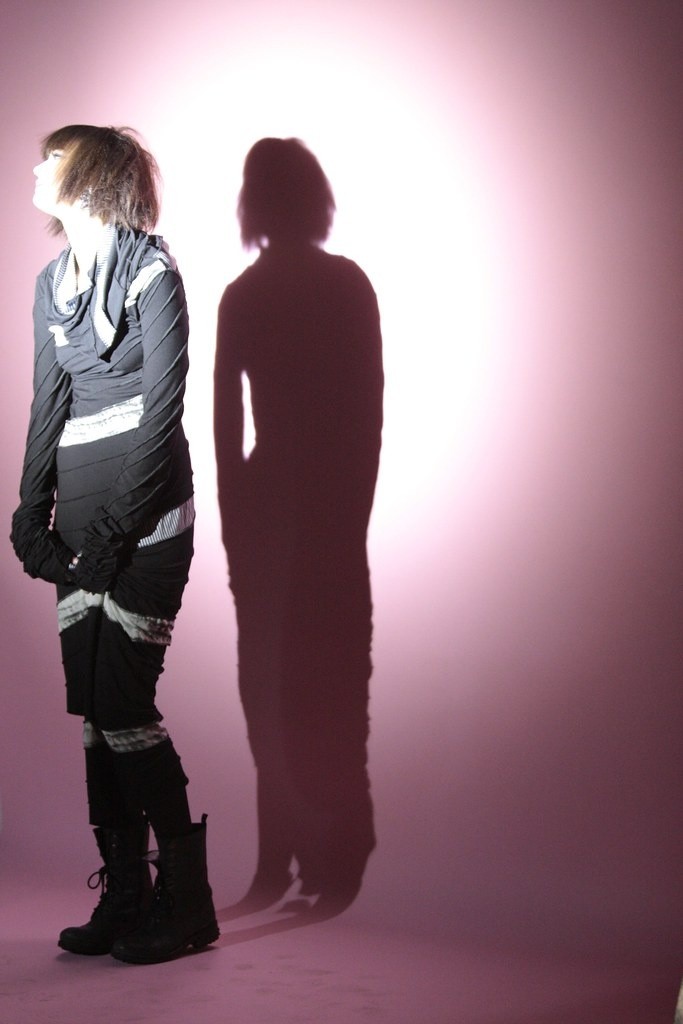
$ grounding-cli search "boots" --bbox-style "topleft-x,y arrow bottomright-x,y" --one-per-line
111,813 -> 220,963
59,819 -> 154,955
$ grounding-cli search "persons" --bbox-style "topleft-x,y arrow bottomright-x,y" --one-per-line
10,125 -> 219,964
